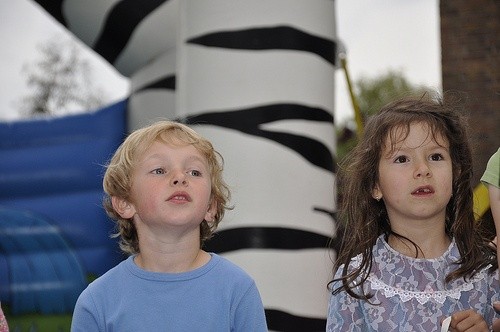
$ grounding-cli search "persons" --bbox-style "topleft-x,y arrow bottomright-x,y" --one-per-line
478,145 -> 500,332
66,120 -> 271,332
323,92 -> 500,332
